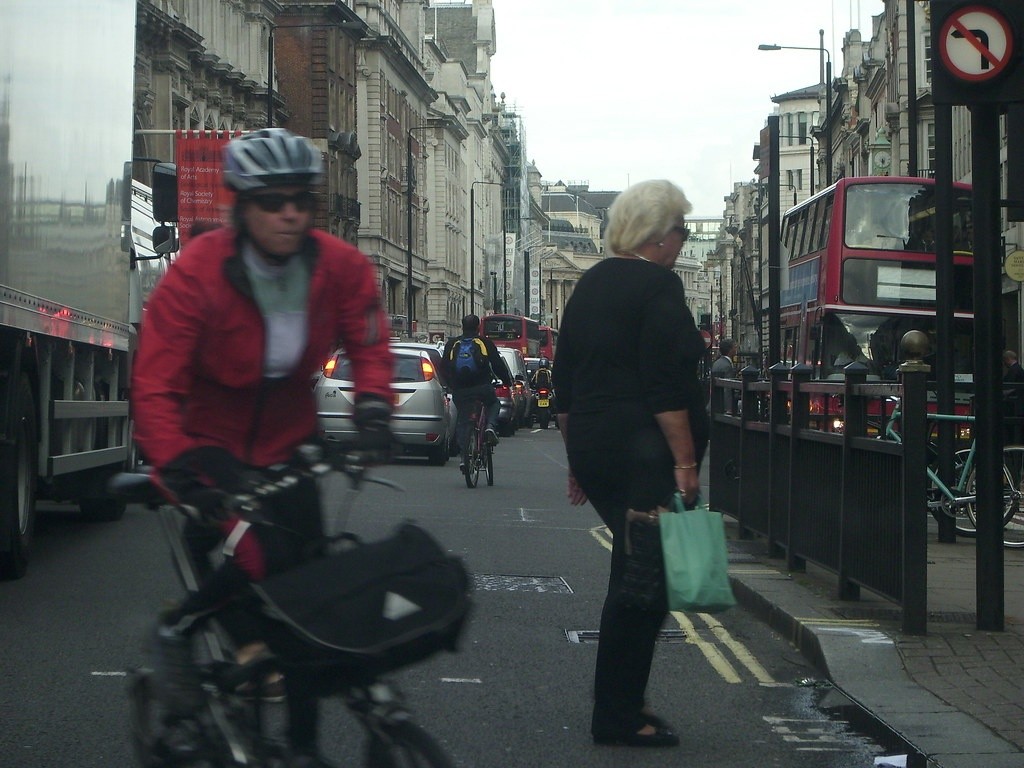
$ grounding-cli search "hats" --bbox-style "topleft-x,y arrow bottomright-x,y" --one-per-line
462,314 -> 479,327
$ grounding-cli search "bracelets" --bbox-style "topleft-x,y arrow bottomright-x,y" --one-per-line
673,463 -> 697,469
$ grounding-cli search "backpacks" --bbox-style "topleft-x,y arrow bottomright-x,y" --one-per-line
447,336 -> 489,389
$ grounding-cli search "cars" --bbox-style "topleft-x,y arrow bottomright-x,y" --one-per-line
312,349 -> 451,466
489,346 -> 541,437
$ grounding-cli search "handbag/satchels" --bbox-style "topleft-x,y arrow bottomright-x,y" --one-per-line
249,524 -> 469,660
627,510 -> 666,583
660,492 -> 735,610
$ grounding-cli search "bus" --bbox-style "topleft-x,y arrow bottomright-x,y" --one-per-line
779,176 -> 972,443
480,314 -> 559,361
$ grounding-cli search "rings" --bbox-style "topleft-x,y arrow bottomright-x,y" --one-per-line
680,489 -> 686,496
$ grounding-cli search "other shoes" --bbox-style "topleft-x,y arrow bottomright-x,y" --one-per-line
485,429 -> 499,446
460,463 -> 465,472
591,725 -> 680,747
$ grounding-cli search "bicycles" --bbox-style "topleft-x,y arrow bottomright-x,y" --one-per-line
446,382 -> 512,489
867,395 -> 1024,549
106,424 -> 473,768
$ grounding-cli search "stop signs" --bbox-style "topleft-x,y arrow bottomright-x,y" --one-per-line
699,330 -> 712,349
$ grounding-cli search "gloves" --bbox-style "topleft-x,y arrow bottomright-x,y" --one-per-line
153,449 -> 235,527
352,401 -> 391,433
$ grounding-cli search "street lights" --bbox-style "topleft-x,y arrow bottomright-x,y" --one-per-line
407,124 -> 456,336
693,280 -> 713,348
563,278 -> 577,311
525,243 -> 556,318
471,181 -> 513,315
267,21 -> 366,128
503,217 -> 537,314
759,44 -> 834,186
539,257 -> 564,326
550,266 -> 571,328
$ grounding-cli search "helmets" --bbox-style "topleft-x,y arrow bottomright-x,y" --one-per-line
539,357 -> 549,368
222,127 -> 323,193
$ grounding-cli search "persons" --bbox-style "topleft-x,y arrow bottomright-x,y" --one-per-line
132,128 -> 396,758
553,179 -> 711,746
712,339 -> 737,378
440,314 -> 515,474
528,358 -> 559,427
1003,350 -> 1024,383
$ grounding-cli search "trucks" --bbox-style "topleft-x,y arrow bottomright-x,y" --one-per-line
0,0 -> 179,581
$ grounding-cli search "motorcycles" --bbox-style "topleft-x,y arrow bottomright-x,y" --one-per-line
531,386 -> 556,429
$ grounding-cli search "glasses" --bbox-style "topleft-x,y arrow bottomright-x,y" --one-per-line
244,191 -> 316,213
674,227 -> 691,241
